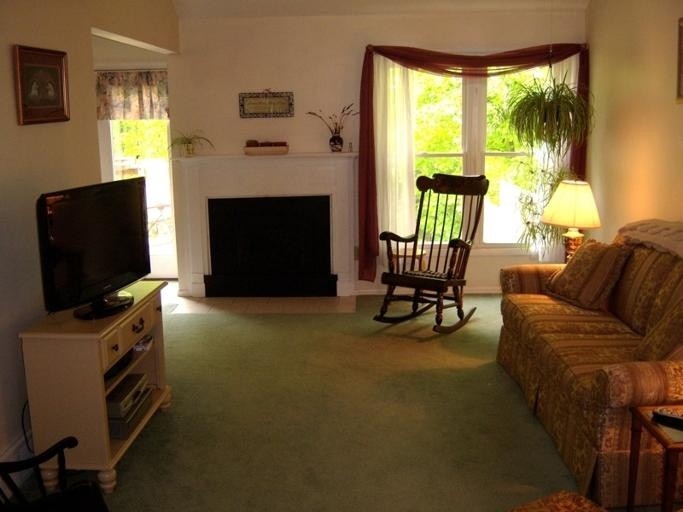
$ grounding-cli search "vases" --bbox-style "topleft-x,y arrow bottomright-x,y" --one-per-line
329,135 -> 346,153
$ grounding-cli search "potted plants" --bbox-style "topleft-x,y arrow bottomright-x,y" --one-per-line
167,128 -> 215,156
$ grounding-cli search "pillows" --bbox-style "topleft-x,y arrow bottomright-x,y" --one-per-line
543,239 -> 633,313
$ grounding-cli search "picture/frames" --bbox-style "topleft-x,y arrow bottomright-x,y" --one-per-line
237,92 -> 296,120
14,45 -> 71,127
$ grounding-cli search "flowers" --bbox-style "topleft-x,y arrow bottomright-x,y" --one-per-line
307,103 -> 360,138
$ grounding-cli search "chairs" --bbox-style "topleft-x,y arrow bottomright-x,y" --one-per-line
373,173 -> 490,334
1,436 -> 109,512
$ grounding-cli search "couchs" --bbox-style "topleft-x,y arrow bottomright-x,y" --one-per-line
495,217 -> 683,509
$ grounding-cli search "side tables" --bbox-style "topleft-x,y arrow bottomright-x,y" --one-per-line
627,406 -> 682,512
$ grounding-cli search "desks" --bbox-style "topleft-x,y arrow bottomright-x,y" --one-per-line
16,278 -> 173,496
170,155 -> 355,297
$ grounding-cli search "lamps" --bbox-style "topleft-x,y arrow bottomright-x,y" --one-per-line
541,181 -> 605,266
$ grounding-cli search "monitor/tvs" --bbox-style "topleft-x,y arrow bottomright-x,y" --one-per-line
35,177 -> 151,322
